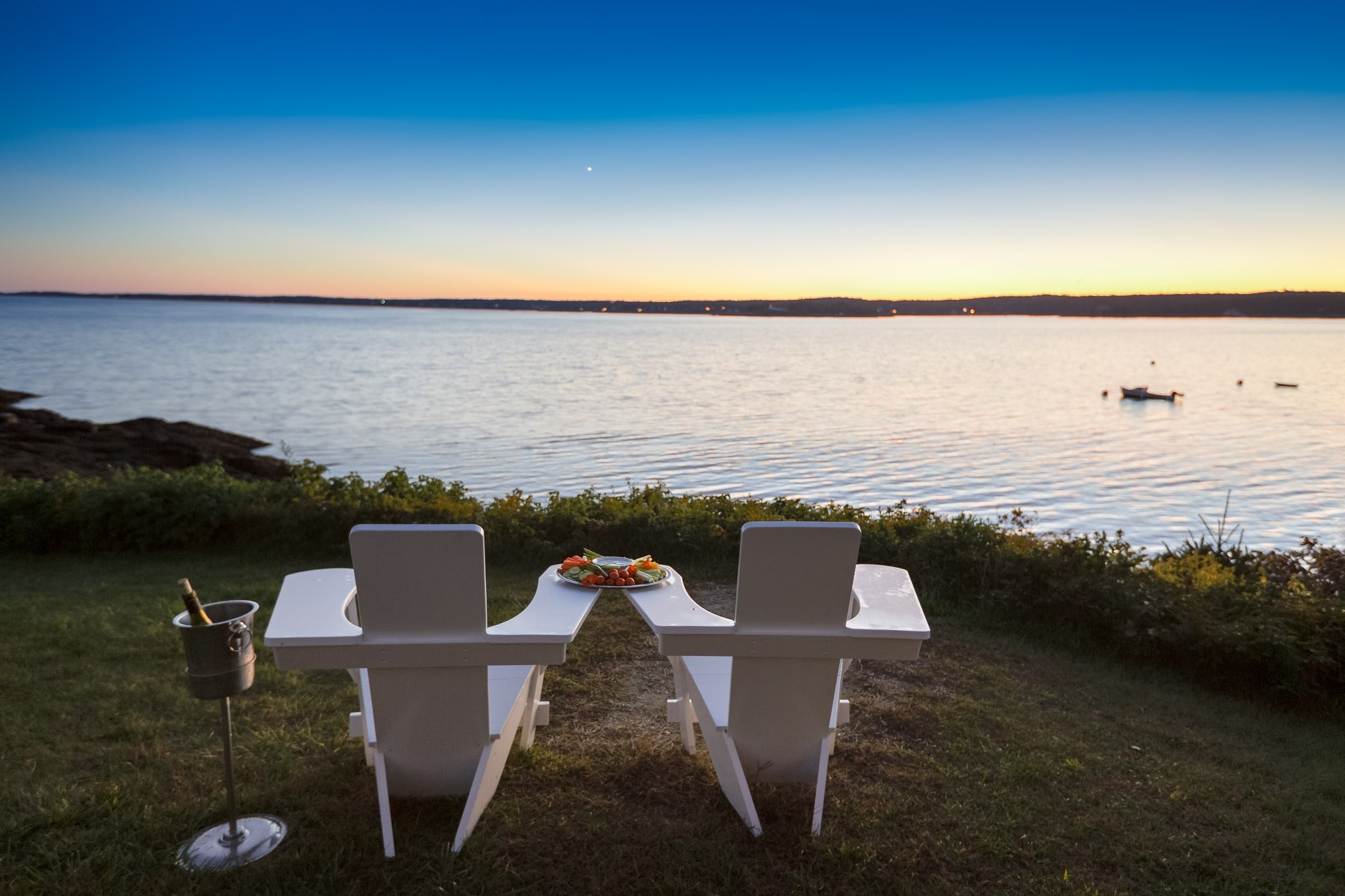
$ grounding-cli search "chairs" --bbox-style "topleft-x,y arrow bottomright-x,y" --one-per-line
264,524 -> 604,857
621,521 -> 931,838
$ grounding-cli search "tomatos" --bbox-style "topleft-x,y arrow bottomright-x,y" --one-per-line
596,569 -> 636,586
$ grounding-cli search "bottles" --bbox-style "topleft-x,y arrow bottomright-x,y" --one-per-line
178,578 -> 212,625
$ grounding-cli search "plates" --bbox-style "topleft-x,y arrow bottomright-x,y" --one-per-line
556,565 -> 671,588
592,556 -> 633,571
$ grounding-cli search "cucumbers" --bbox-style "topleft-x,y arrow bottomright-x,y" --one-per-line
562,566 -> 595,581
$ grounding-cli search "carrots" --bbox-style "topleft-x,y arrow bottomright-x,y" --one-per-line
557,555 -> 590,572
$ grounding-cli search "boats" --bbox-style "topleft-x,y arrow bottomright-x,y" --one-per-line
1274,381 -> 1299,388
1119,384 -> 1185,403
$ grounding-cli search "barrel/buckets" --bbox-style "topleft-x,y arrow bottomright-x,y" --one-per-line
173,599 -> 259,698
